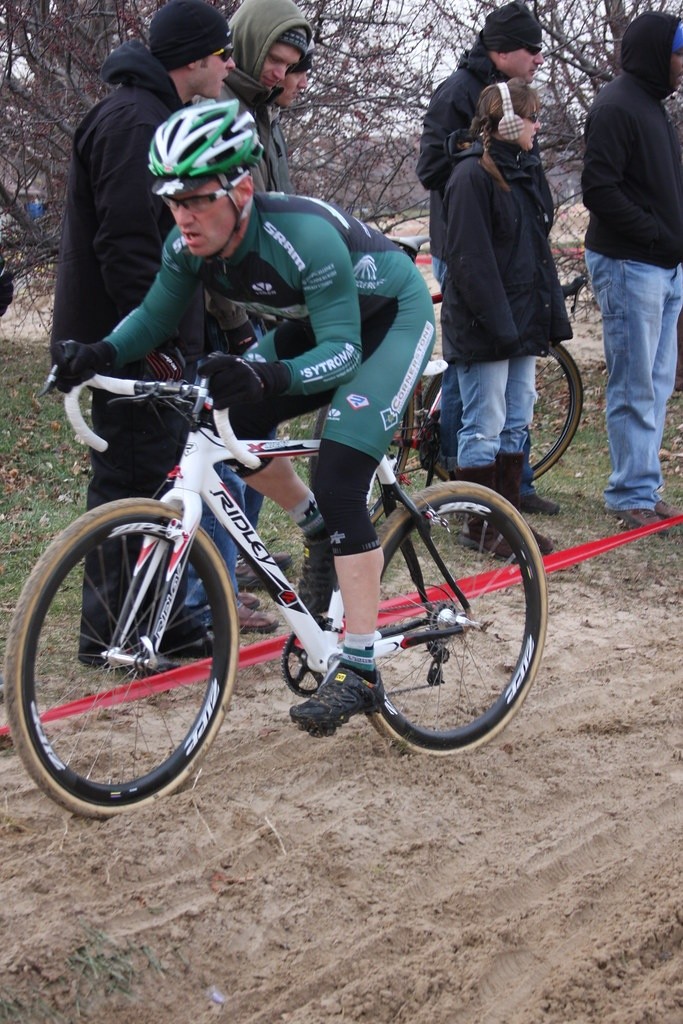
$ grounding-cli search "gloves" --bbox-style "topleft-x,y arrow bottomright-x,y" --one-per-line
49,341 -> 112,393
196,353 -> 288,410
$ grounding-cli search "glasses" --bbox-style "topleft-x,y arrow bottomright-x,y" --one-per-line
213,48 -> 234,61
494,26 -> 542,54
159,167 -> 250,210
527,111 -> 538,123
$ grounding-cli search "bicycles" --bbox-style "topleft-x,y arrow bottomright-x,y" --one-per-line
307,238 -> 584,524
3,341 -> 550,823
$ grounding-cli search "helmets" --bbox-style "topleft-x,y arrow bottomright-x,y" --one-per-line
146,96 -> 264,198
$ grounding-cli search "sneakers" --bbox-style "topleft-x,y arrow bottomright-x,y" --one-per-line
297,534 -> 339,616
288,668 -> 385,730
520,493 -> 560,516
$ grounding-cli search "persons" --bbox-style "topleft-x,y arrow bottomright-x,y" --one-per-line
440,79 -> 574,564
583,11 -> 683,530
51,96 -> 440,737
415,2 -> 561,516
49,0 -> 319,664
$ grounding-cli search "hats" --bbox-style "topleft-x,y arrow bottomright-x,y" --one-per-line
148,0 -> 233,73
671,20 -> 683,53
482,1 -> 543,53
286,38 -> 315,73
274,27 -> 308,62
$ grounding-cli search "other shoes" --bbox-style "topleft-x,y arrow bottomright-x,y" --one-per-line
160,630 -> 216,658
78,638 -> 180,677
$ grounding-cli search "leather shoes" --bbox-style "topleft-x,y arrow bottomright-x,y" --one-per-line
201,596 -> 278,634
234,561 -> 267,588
268,552 -> 291,570
236,592 -> 260,609
605,501 -> 671,536
655,500 -> 682,519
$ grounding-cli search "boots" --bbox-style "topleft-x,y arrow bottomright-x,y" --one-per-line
495,452 -> 554,553
454,464 -> 534,564
